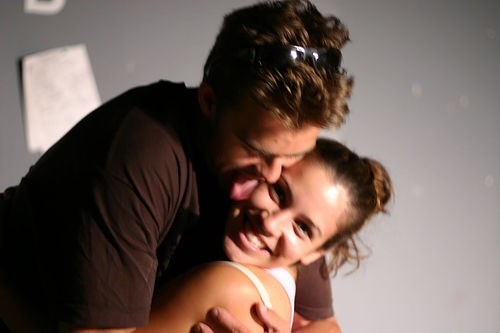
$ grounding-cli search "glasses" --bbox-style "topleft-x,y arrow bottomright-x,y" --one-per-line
253,41 -> 342,72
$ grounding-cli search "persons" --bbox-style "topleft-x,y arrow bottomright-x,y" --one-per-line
0,0 -> 354,333
132,137 -> 396,333
